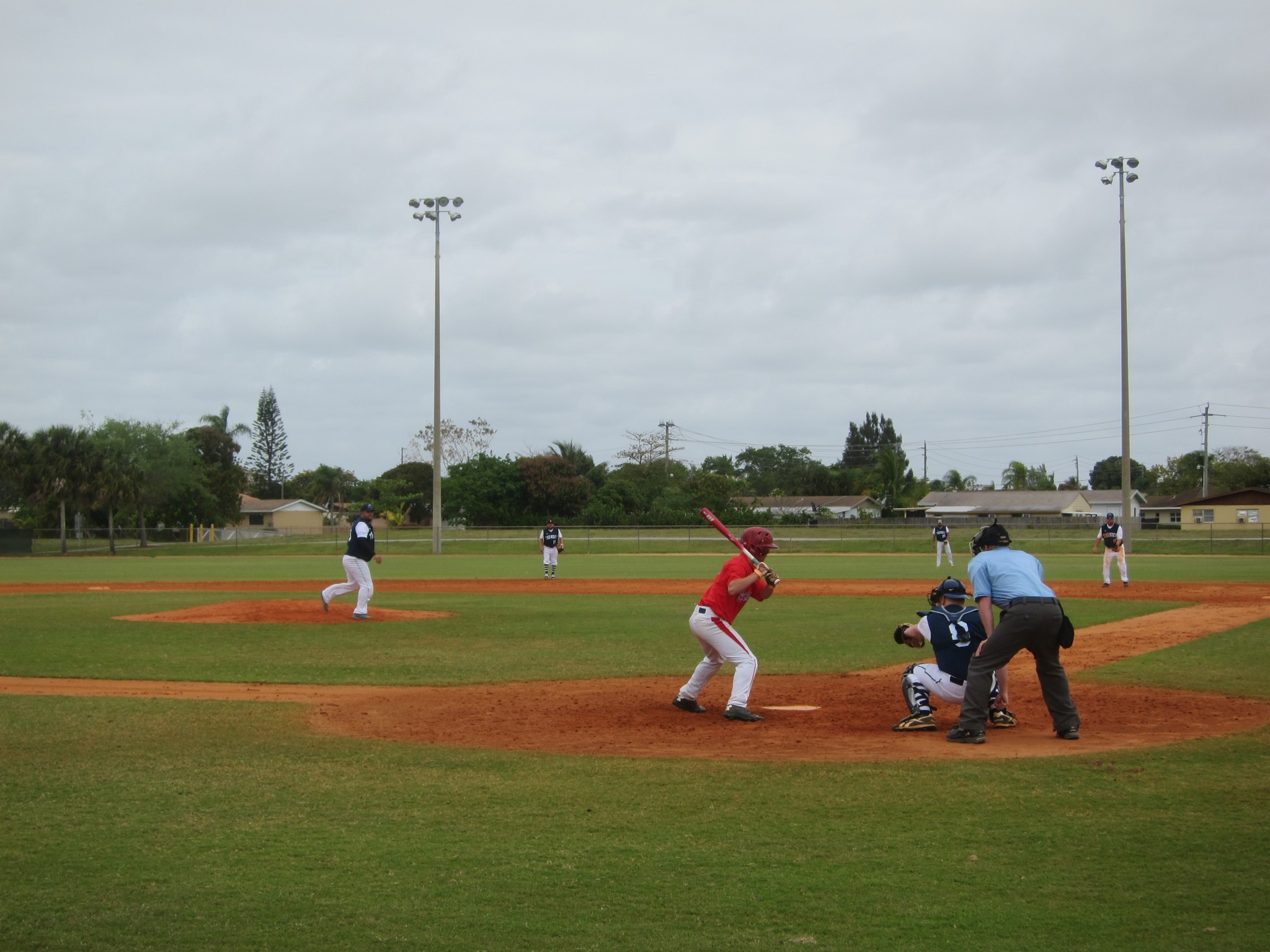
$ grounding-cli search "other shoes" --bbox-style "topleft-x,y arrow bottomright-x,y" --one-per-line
1102,583 -> 1110,587
950,563 -> 954,567
1123,581 -> 1128,587
936,565 -> 941,568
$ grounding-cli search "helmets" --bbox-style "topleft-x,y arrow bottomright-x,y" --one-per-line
738,527 -> 779,555
935,579 -> 971,601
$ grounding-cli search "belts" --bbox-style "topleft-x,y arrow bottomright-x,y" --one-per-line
698,607 -> 707,614
949,677 -> 967,687
1010,595 -> 1059,607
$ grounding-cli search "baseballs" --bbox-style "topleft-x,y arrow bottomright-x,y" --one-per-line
670,473 -> 674,478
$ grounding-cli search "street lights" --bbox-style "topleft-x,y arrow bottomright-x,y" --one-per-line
403,198 -> 468,555
1091,156 -> 1142,542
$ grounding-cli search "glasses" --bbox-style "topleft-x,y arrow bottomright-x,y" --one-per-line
1107,518 -> 1113,520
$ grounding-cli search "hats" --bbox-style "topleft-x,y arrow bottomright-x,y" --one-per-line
360,504 -> 375,513
938,519 -> 942,524
978,518 -> 1012,550
546,519 -> 554,524
1107,512 -> 1114,519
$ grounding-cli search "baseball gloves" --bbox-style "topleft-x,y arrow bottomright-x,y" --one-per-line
1110,544 -> 1120,552
894,623 -> 925,649
557,545 -> 564,553
943,540 -> 948,546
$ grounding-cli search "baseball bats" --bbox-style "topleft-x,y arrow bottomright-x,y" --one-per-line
700,507 -> 780,585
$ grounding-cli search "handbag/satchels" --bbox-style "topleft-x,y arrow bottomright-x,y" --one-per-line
1059,615 -> 1075,649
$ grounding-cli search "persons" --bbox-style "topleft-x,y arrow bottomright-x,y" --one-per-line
671,526 -> 779,722
1094,513 -> 1128,587
321,503 -> 384,619
540,519 -> 565,580
891,576 -> 1018,732
931,519 -> 954,567
946,519 -> 1082,743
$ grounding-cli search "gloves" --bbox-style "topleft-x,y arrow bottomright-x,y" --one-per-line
754,561 -> 770,578
765,568 -> 779,588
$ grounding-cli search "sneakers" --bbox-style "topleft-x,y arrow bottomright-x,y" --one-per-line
544,574 -> 549,579
987,707 -> 1018,728
551,574 -> 555,579
351,613 -> 373,620
891,710 -> 938,732
722,704 -> 765,722
672,692 -> 707,713
321,590 -> 330,611
946,723 -> 986,744
1056,726 -> 1080,740
